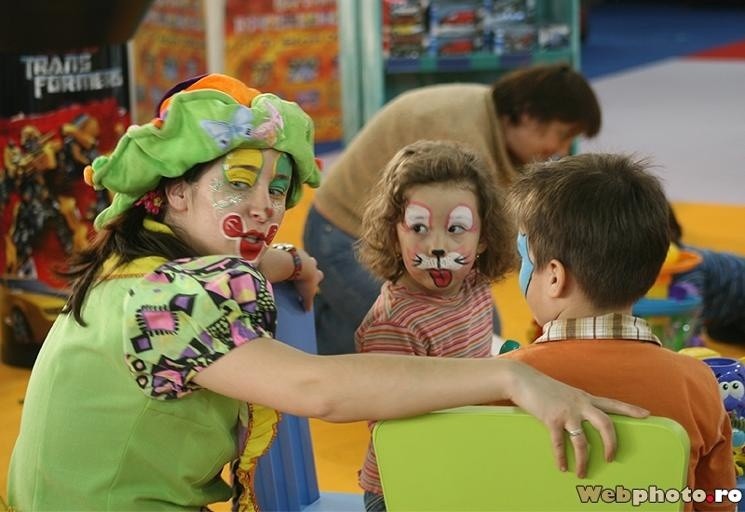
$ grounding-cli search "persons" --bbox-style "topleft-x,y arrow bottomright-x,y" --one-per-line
8,62 -> 744,512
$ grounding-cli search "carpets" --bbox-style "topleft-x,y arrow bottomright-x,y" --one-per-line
1,200 -> 745,511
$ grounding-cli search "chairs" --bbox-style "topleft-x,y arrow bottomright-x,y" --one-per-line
254,278 -> 365,512
369,403 -> 690,510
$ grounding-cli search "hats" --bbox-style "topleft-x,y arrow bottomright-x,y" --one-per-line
84,71 -> 322,231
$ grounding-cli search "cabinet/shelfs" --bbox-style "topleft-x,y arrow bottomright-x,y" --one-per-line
361,0 -> 583,155
